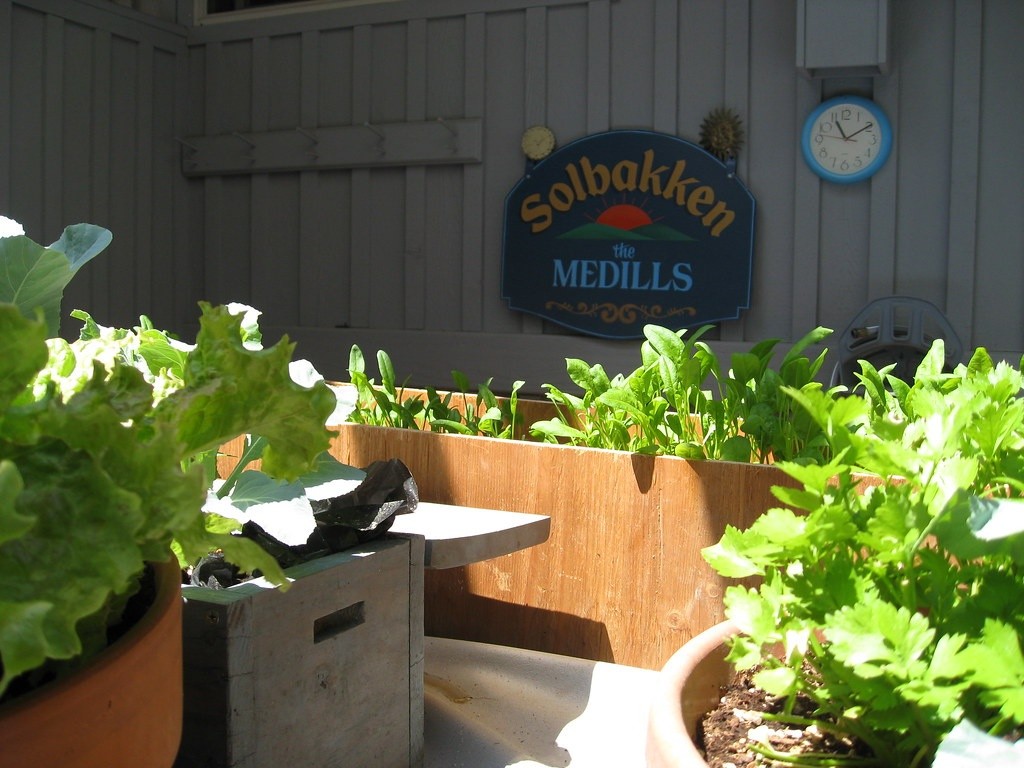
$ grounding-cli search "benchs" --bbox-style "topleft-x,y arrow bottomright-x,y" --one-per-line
398,500 -> 558,567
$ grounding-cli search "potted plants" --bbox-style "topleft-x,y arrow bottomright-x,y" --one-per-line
0,205 -> 1024,768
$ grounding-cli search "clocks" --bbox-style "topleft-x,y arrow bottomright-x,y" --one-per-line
800,95 -> 893,183
522,124 -> 556,160
